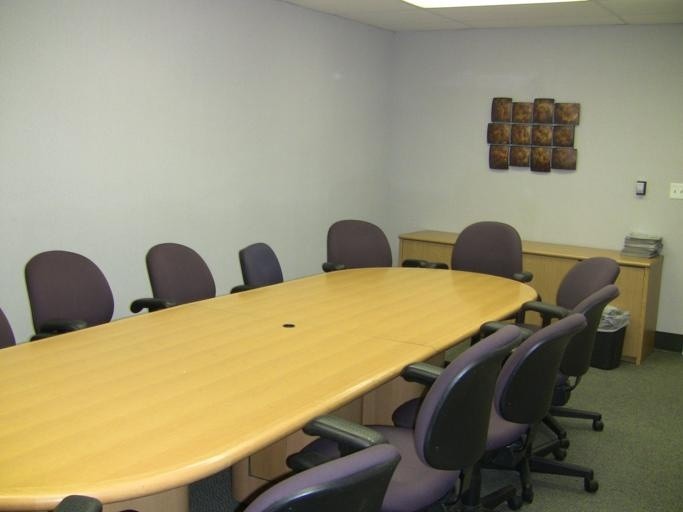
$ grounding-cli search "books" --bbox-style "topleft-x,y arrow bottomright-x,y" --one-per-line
619,232 -> 663,260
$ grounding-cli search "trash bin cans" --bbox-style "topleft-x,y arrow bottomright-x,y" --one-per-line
593,306 -> 629,369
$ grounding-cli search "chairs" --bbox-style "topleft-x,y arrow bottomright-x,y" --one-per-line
235,257 -> 622,511
451,221 -> 533,284
1,310 -> 19,351
23,248 -> 115,342
230,242 -> 284,296
129,242 -> 216,317
321,220 -> 392,273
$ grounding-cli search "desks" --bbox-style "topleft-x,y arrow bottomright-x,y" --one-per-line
3,264 -> 540,511
395,229 -> 665,365
